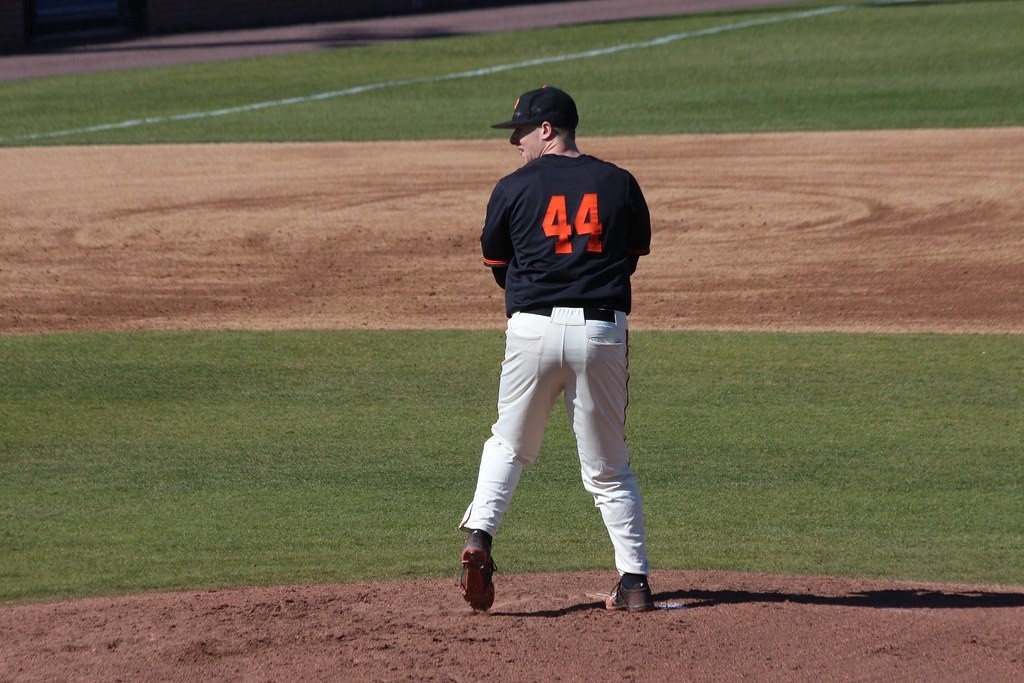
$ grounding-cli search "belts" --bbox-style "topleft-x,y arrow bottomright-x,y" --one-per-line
521,305 -> 615,324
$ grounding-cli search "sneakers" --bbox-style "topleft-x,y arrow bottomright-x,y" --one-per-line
460,531 -> 498,611
605,580 -> 653,612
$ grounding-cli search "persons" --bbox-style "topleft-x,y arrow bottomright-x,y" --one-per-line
458,85 -> 656,613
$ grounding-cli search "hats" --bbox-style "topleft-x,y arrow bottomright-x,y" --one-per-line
491,85 -> 578,129
